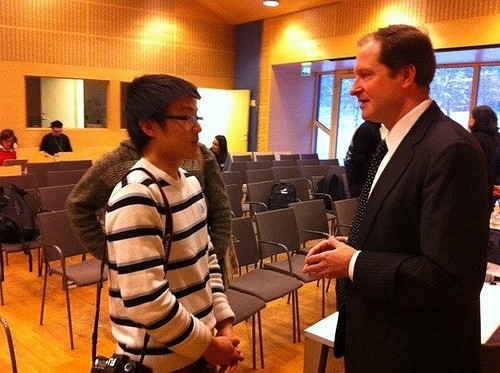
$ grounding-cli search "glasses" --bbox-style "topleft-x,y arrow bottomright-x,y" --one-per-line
212,141 -> 219,146
54,130 -> 61,133
167,114 -> 204,126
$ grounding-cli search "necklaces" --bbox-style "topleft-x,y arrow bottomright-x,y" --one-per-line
53,133 -> 63,152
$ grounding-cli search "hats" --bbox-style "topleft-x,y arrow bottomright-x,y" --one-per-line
50,120 -> 62,128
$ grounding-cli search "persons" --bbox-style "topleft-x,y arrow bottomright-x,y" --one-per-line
105,74 -> 244,373
303,26 -> 500,373
66,138 -> 233,268
39,120 -> 73,156
0,128 -> 18,166
209,135 -> 232,172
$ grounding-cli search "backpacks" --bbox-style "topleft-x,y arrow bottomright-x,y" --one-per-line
268,181 -> 299,210
0,184 -> 41,243
315,170 -> 348,210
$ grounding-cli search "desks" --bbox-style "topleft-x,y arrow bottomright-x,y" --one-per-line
303,282 -> 500,373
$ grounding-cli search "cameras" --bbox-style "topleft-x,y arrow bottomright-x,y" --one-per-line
91,353 -> 152,373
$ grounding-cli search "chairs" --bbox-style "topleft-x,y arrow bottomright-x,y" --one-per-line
0,153 -> 360,373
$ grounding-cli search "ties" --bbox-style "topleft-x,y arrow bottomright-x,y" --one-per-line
335,139 -> 388,311
